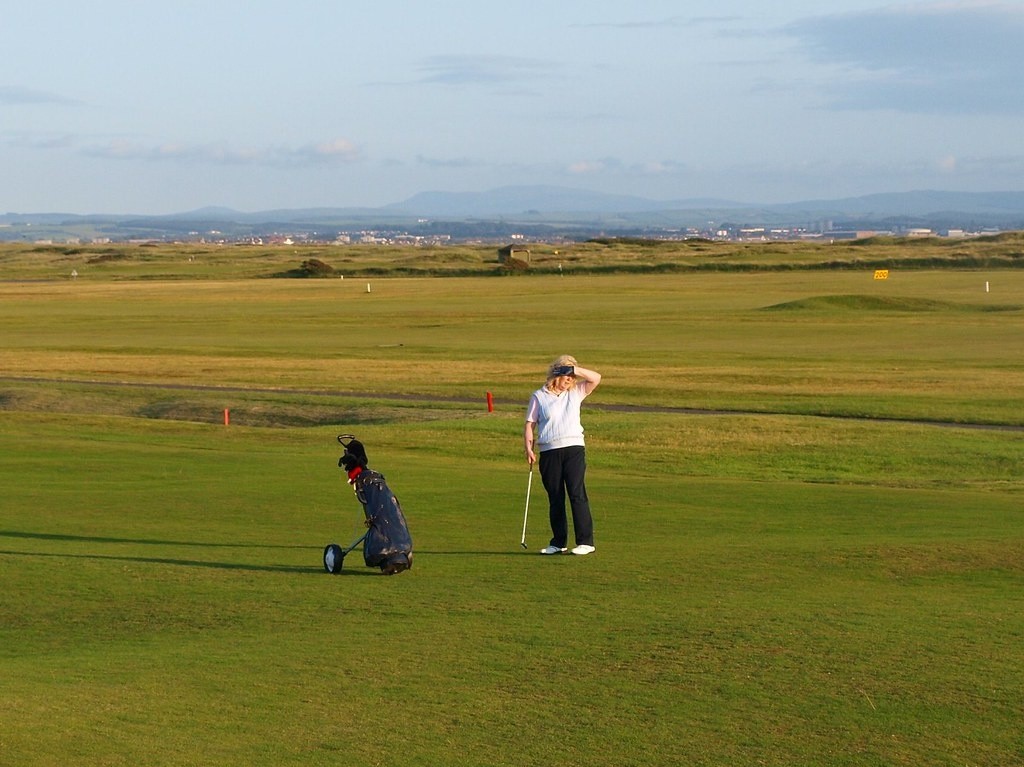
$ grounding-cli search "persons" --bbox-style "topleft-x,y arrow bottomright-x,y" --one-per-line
524,355 -> 602,554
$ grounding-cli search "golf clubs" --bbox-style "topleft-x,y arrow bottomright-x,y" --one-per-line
520,439 -> 536,550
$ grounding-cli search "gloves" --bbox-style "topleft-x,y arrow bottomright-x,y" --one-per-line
552,366 -> 574,375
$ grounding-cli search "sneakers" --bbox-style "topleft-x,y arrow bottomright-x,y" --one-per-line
571,544 -> 595,554
540,545 -> 568,554
